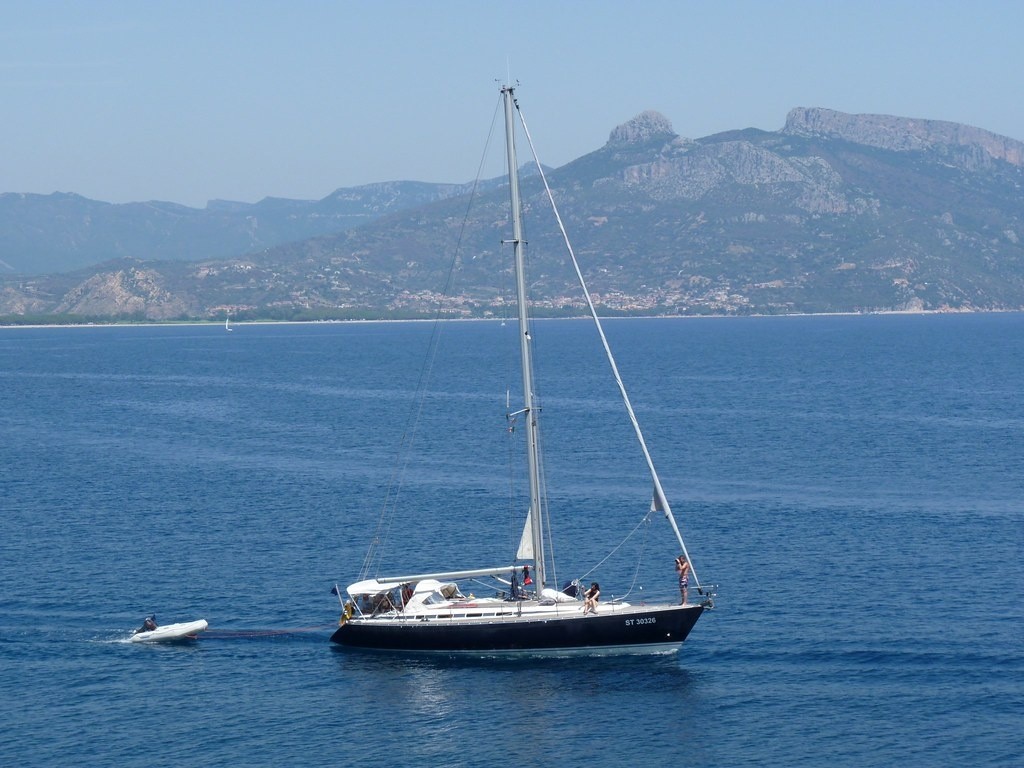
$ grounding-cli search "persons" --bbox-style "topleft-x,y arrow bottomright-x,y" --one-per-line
362,583 -> 413,614
143,617 -> 157,631
675,555 -> 689,606
580,582 -> 600,615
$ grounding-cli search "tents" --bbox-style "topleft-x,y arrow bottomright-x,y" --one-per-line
346,579 -> 413,619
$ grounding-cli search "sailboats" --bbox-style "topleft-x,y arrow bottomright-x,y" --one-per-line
326,77 -> 720,656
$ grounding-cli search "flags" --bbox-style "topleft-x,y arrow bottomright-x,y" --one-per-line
331,586 -> 343,596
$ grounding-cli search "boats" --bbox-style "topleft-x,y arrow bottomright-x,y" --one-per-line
128,618 -> 209,643
